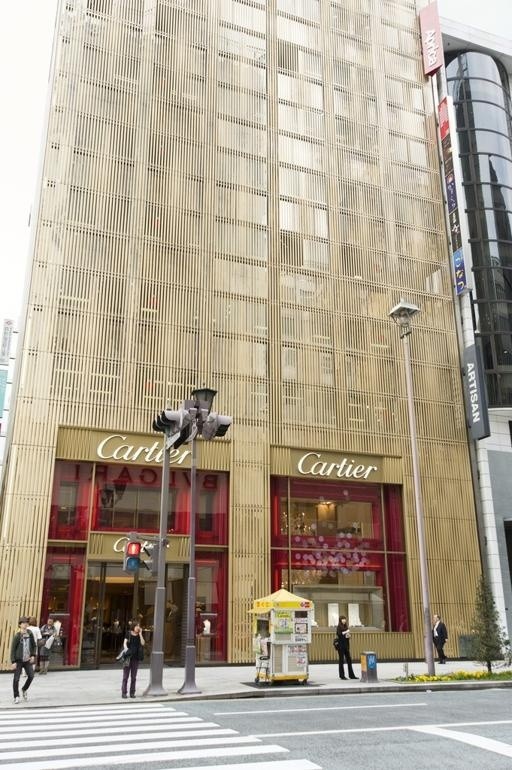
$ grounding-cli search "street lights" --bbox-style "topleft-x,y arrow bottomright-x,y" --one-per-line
191,382 -> 218,433
388,298 -> 435,677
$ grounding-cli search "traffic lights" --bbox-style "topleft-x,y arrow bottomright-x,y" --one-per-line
152,409 -> 180,436
204,414 -> 231,441
123,540 -> 157,575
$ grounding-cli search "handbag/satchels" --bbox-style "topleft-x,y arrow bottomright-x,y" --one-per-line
334,638 -> 341,651
115,651 -> 125,664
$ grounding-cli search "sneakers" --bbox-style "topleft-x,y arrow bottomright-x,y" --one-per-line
14,696 -> 20,704
22,688 -> 28,699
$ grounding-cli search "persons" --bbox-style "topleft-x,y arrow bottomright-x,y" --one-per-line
11,617 -> 36,703
433,614 -> 447,664
38,616 -> 57,674
334,617 -> 358,680
121,621 -> 146,698
27,617 -> 43,674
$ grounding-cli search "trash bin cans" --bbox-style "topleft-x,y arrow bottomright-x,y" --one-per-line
361,652 -> 377,682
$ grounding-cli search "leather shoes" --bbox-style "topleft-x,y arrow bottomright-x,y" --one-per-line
130,693 -> 135,698
122,693 -> 127,698
350,676 -> 358,679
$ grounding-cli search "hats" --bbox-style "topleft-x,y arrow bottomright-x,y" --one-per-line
18,617 -> 28,624
